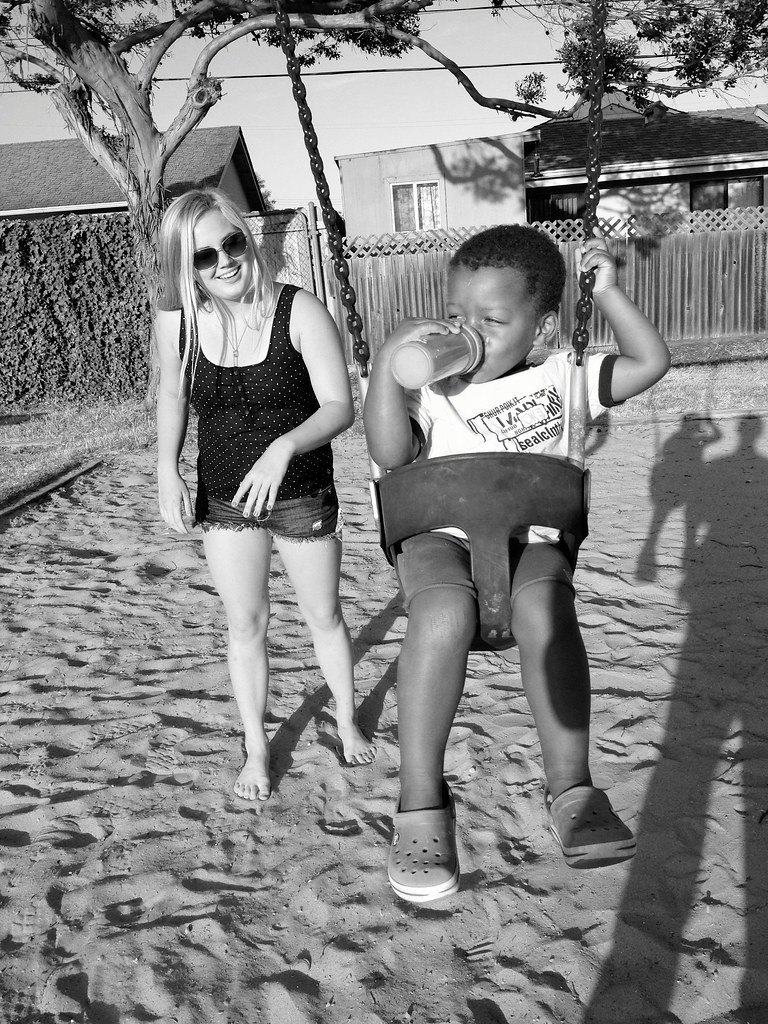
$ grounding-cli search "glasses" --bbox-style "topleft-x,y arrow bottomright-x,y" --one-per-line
194,231 -> 248,270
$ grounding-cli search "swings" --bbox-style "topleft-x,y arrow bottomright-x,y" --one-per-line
269,1 -> 610,652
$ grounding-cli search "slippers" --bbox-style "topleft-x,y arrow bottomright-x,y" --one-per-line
385,778 -> 461,903
542,787 -> 638,867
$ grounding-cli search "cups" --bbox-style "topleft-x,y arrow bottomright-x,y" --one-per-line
391,322 -> 483,390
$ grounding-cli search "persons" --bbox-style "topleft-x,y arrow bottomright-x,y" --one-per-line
354,221 -> 671,904
151,191 -> 375,804
